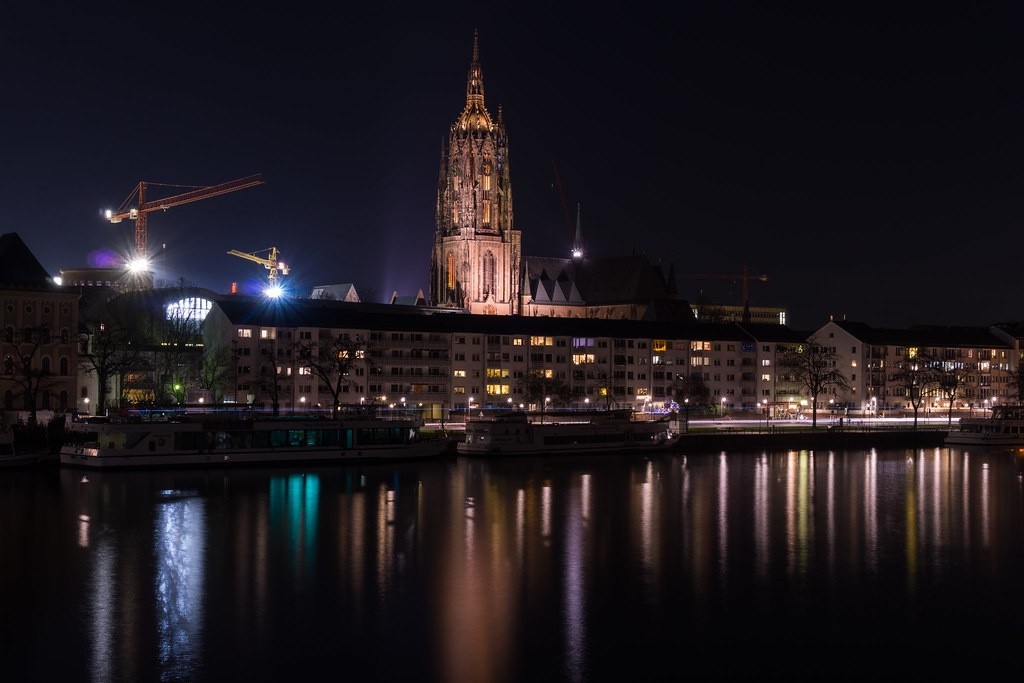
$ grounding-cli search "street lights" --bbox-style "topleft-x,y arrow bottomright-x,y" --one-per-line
984,399 -> 990,418
720,398 -> 726,421
757,403 -> 762,433
763,399 -> 768,428
788,398 -> 793,419
292,392 -> 557,424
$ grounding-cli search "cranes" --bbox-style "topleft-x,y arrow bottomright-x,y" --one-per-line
227,248 -> 289,294
109,172 -> 269,272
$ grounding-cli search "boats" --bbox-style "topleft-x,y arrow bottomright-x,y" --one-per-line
944,406 -> 1024,444
456,406 -> 682,455
60,410 -> 449,468
